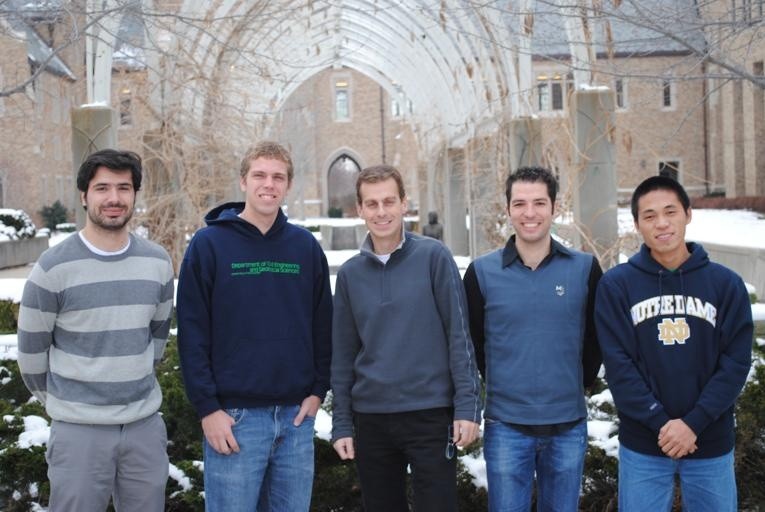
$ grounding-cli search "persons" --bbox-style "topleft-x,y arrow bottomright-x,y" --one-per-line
331,165 -> 482,511
594,175 -> 754,512
464,168 -> 604,512
177,142 -> 330,512
422,211 -> 443,240
18,149 -> 174,511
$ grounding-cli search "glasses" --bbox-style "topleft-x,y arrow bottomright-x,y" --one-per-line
444,423 -> 457,462
